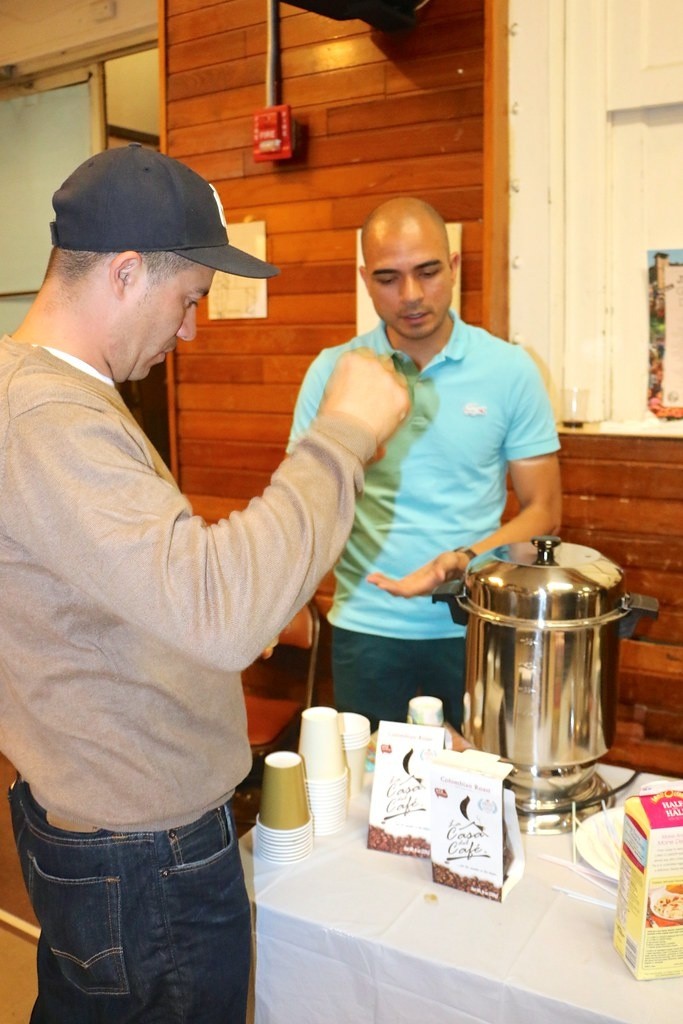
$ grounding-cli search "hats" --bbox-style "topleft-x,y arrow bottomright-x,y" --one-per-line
49,142 -> 282,280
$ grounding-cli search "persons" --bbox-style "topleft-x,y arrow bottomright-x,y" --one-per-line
257,198 -> 562,736
0,143 -> 409,1024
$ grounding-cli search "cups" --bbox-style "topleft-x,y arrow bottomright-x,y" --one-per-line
256,696 -> 452,863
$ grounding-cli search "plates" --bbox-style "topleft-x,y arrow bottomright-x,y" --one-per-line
573,807 -> 630,882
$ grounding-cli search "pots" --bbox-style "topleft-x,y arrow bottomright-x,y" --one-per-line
430,535 -> 659,834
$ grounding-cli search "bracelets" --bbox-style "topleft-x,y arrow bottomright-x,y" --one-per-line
453,547 -> 476,561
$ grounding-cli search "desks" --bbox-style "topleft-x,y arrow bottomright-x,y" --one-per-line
240,729 -> 681,1024
